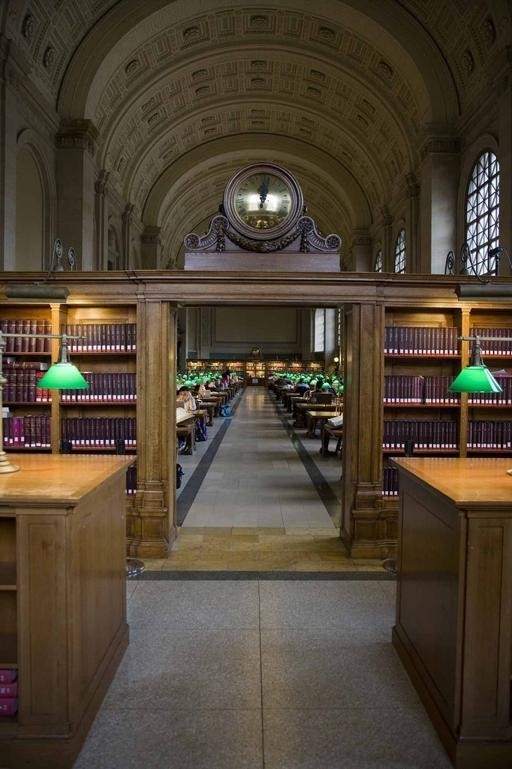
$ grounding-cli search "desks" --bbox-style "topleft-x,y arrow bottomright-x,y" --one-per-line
391,457 -> 512,769
0,454 -> 138,769
176,380 -> 243,455
268,379 -> 343,462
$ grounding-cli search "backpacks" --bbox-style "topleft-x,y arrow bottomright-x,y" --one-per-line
219,404 -> 230,417
194,419 -> 209,442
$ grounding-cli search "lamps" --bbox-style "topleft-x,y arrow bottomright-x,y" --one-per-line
448,335 -> 512,392
0,331 -> 90,390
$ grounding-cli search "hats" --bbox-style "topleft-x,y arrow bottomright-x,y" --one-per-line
178,385 -> 191,393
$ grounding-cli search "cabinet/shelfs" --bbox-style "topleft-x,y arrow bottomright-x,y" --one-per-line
1,303 -> 138,494
377,303 -> 511,504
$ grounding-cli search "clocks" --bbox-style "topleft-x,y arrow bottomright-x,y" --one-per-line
183,161 -> 342,271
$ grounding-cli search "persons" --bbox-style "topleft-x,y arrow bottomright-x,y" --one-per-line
268,371 -> 344,440
174,368 -> 245,454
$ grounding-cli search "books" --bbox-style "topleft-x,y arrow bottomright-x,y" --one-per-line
0,668 -> 19,683
0,682 -> 18,699
0,698 -> 18,718
380,325 -> 510,500
0,317 -> 137,499
